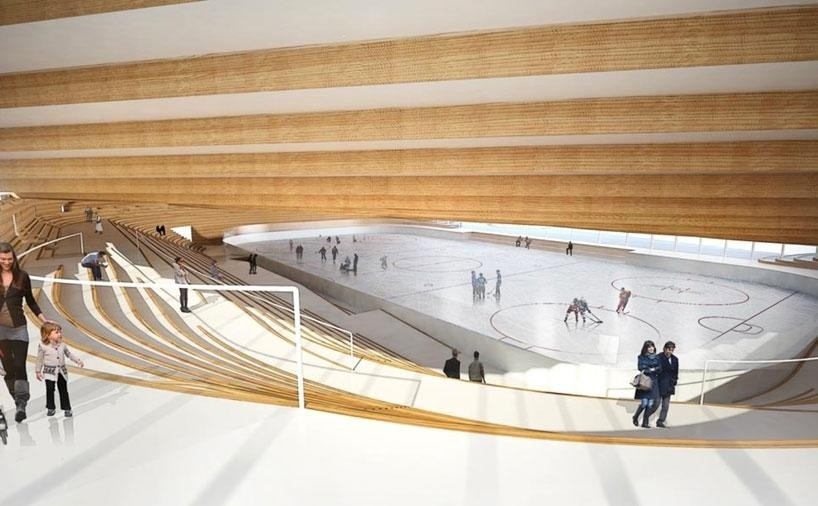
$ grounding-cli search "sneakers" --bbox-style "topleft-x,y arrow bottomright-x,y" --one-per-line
65,410 -> 72,416
47,409 -> 55,416
633,416 -> 665,428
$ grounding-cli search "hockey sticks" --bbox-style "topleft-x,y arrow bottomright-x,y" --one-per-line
584,309 -> 603,323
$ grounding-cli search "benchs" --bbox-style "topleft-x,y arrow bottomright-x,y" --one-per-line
0,197 -> 444,430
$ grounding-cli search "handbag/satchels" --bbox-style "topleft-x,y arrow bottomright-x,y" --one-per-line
633,370 -> 652,392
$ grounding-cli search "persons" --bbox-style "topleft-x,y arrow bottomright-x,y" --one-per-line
248,252 -> 258,274
285,232 -> 359,274
567,241 -> 573,258
443,348 -> 460,380
565,297 -> 580,321
468,350 -> 485,383
580,298 -> 593,321
0,243 -> 57,422
209,259 -> 224,282
173,256 -> 192,313
83,205 -> 106,234
36,322 -> 84,417
525,236 -> 533,247
155,225 -> 166,236
634,340 -> 662,428
468,269 -> 502,300
648,341 -> 678,429
615,287 -> 631,312
75,250 -> 108,281
516,236 -> 522,247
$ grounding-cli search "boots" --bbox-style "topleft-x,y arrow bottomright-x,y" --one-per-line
4,377 -> 30,422
